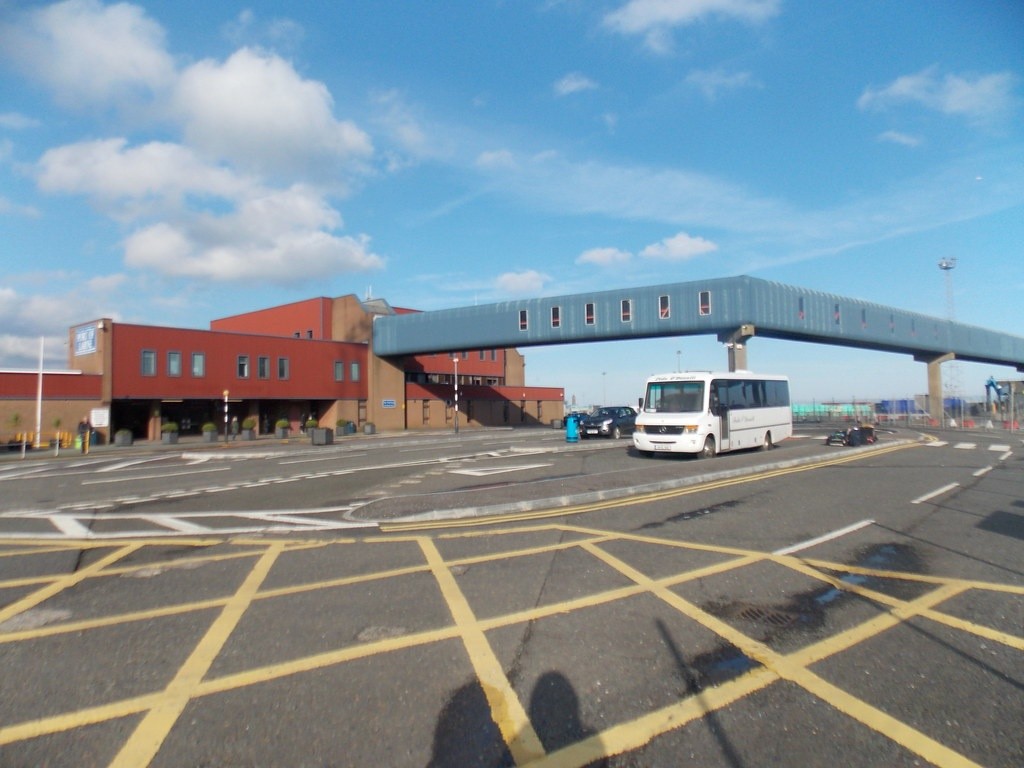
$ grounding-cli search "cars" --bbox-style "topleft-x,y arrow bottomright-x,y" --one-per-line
563,411 -> 590,428
579,406 -> 637,440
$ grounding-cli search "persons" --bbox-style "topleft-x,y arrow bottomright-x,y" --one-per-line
230,415 -> 239,440
79,416 -> 92,455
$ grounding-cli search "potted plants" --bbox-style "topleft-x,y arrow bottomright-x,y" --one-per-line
306,419 -> 318,438
337,419 -> 346,437
275,418 -> 289,438
201,422 -> 218,443
160,423 -> 178,445
362,421 -> 375,435
240,418 -> 257,441
115,429 -> 133,445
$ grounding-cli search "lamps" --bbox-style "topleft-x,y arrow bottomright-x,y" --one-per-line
98,319 -> 105,331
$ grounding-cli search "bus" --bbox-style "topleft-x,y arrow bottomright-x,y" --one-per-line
632,370 -> 792,460
